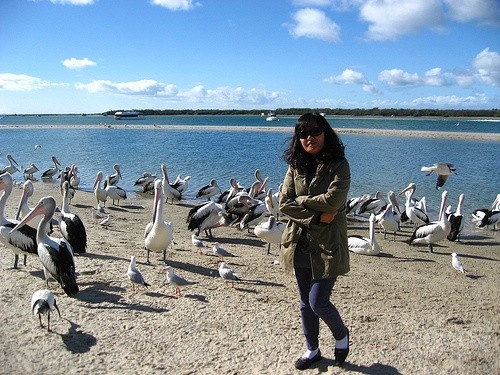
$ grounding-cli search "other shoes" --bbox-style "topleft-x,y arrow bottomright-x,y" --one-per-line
295,348 -> 321,369
334,328 -> 349,364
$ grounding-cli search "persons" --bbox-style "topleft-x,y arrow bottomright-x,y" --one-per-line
279,113 -> 350,371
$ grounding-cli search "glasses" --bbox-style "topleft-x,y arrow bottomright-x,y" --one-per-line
298,126 -> 324,139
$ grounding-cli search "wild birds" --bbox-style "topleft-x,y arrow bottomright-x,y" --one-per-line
219,261 -> 244,289
163,265 -> 200,295
211,241 -> 238,261
127,255 -> 151,290
90,208 -> 103,224
345,161 -> 500,257
0,172 -> 87,297
30,289 -> 61,331
191,235 -> 208,253
142,180 -> 174,266
34,144 -> 41,150
186,167 -> 284,254
99,122 -> 127,128
99,214 -> 114,230
133,163 -> 189,204
97,204 -> 105,213
0,153 -> 81,190
153,122 -> 157,126
91,164 -> 127,209
450,253 -> 466,275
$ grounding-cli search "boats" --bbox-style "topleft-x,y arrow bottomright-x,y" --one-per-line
114,111 -> 146,120
104,113 -> 107,116
266,111 -> 279,121
320,112 -> 326,116
82,113 -> 87,116
